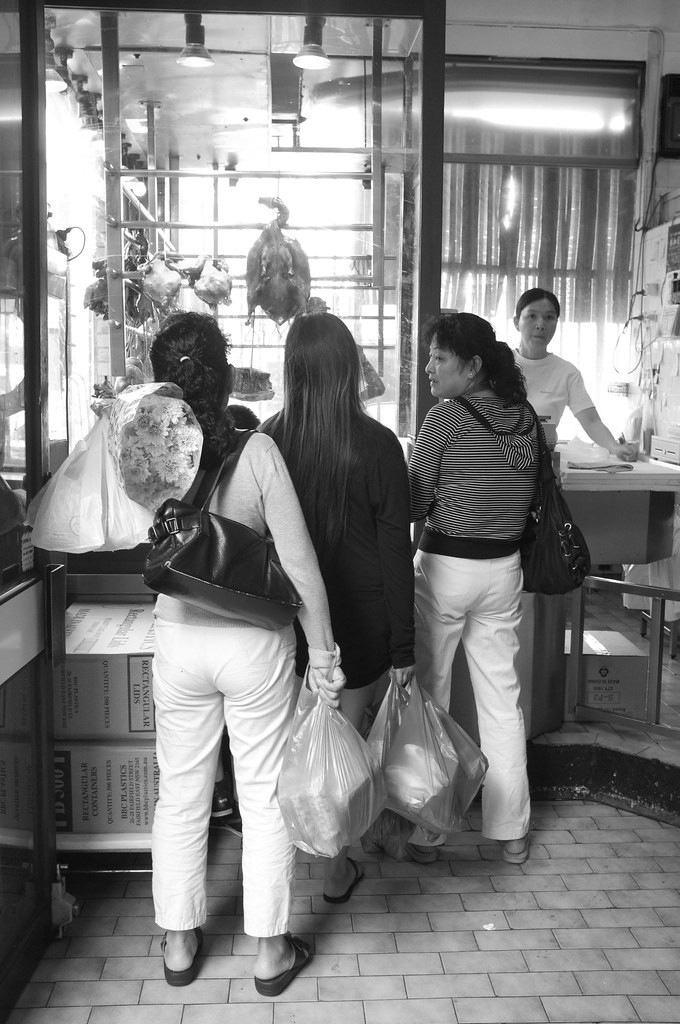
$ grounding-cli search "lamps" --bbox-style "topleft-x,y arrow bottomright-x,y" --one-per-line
292,17 -> 332,70
174,13 -> 217,66
43,14 -> 148,198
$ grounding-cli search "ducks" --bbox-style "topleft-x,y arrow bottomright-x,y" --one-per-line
186,251 -> 232,306
245,197 -> 311,326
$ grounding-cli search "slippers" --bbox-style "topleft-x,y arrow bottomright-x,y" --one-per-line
501,833 -> 529,864
400,832 -> 438,863
253,934 -> 312,996
322,859 -> 364,905
161,924 -> 203,987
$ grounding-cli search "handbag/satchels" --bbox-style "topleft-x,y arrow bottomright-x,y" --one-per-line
140,433 -> 300,630
278,657 -> 489,858
519,398 -> 591,595
22,416 -> 154,555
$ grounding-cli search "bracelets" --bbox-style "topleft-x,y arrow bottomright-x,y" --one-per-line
307,641 -> 341,668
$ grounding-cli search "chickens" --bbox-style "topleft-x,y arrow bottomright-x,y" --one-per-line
136,259 -> 181,305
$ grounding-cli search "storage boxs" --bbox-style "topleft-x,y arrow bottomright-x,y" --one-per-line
0,602 -> 169,834
560,628 -> 648,721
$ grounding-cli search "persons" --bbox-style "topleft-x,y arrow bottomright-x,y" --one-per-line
119,310 -> 347,997
254,313 -> 410,904
509,288 -> 641,462
389,313 -> 549,863
